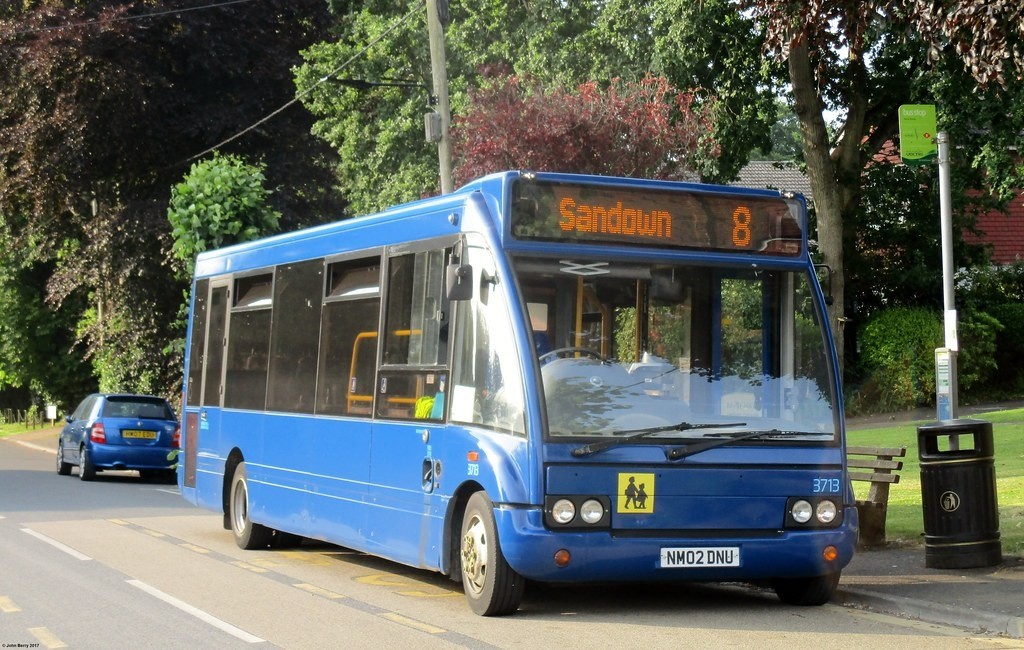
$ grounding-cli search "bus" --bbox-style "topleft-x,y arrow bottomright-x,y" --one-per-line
177,169 -> 861,617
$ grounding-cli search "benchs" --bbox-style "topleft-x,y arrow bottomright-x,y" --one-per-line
845,445 -> 906,549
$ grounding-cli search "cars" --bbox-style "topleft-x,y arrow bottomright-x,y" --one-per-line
58,393 -> 180,481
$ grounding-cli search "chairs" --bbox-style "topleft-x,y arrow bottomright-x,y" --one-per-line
138,406 -> 159,418
197,340 -> 407,420
488,331 -> 560,417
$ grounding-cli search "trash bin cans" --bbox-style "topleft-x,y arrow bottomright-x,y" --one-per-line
916,419 -> 1003,570
